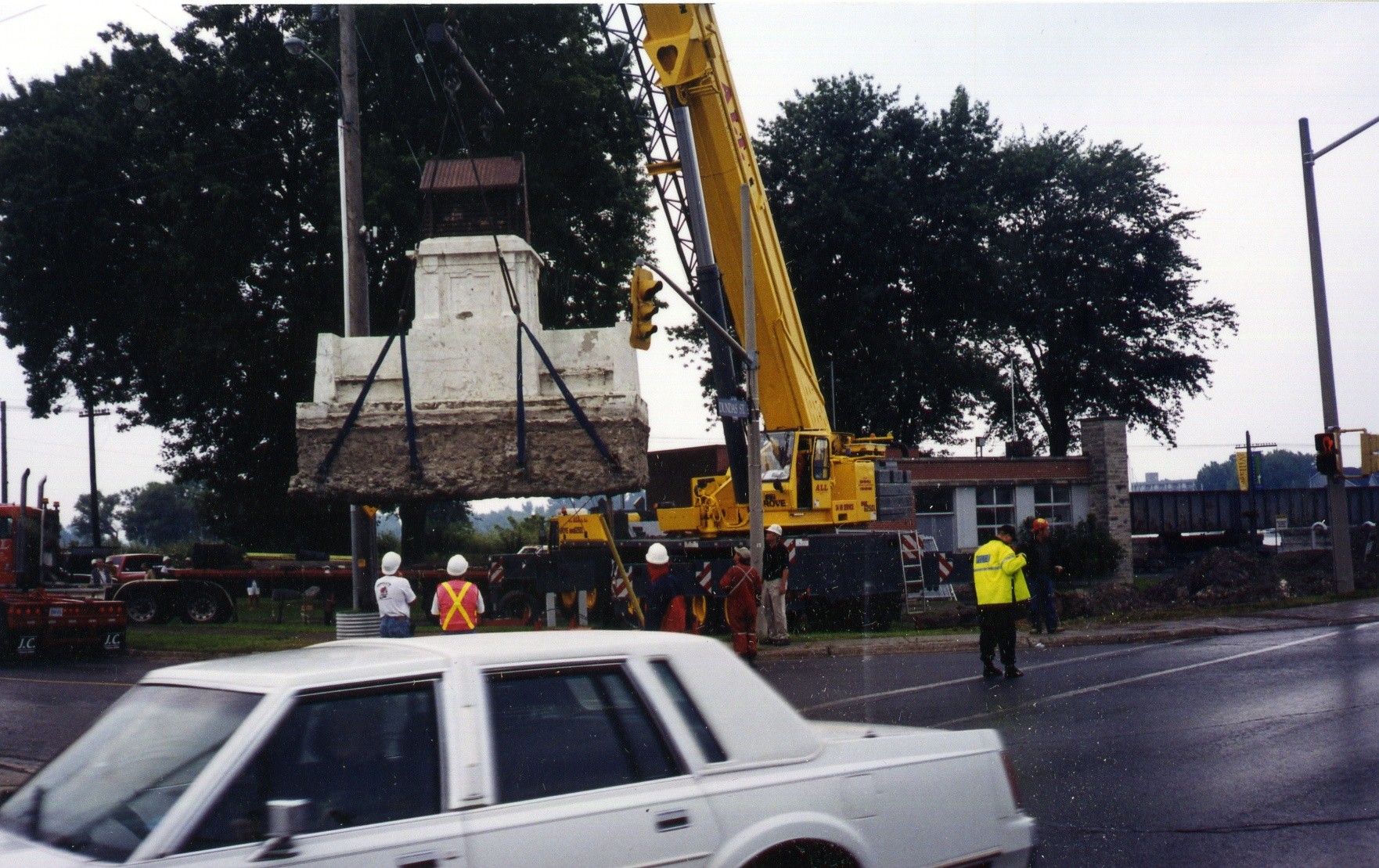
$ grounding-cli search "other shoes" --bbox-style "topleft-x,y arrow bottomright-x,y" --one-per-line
1005,666 -> 1024,678
1030,628 -> 1042,634
1048,626 -> 1066,633
983,663 -> 1003,677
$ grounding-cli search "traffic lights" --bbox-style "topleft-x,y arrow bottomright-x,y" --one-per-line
1314,433 -> 1338,476
628,266 -> 663,352
1361,433 -> 1379,478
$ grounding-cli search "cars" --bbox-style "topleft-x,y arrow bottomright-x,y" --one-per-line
517,545 -> 557,554
0,624 -> 1040,868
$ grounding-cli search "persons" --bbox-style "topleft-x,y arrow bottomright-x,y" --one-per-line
141,562 -> 156,580
245,577 -> 261,611
184,557 -> 194,570
374,551 -> 417,638
161,556 -> 174,573
973,525 -> 1030,679
762,523 -> 790,640
646,543 -> 686,633
89,557 -> 118,587
322,566 -> 337,625
1024,518 -> 1066,634
720,547 -> 762,661
431,554 -> 485,635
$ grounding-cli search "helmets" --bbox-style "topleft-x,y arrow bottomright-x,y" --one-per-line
1032,518 -> 1048,532
645,542 -> 669,564
381,551 -> 401,575
447,554 -> 468,576
765,524 -> 782,536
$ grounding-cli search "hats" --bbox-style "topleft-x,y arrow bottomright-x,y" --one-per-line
734,546 -> 751,559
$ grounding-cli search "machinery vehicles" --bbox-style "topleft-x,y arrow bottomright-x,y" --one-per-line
0,467 -> 234,660
542,3 -> 956,623
